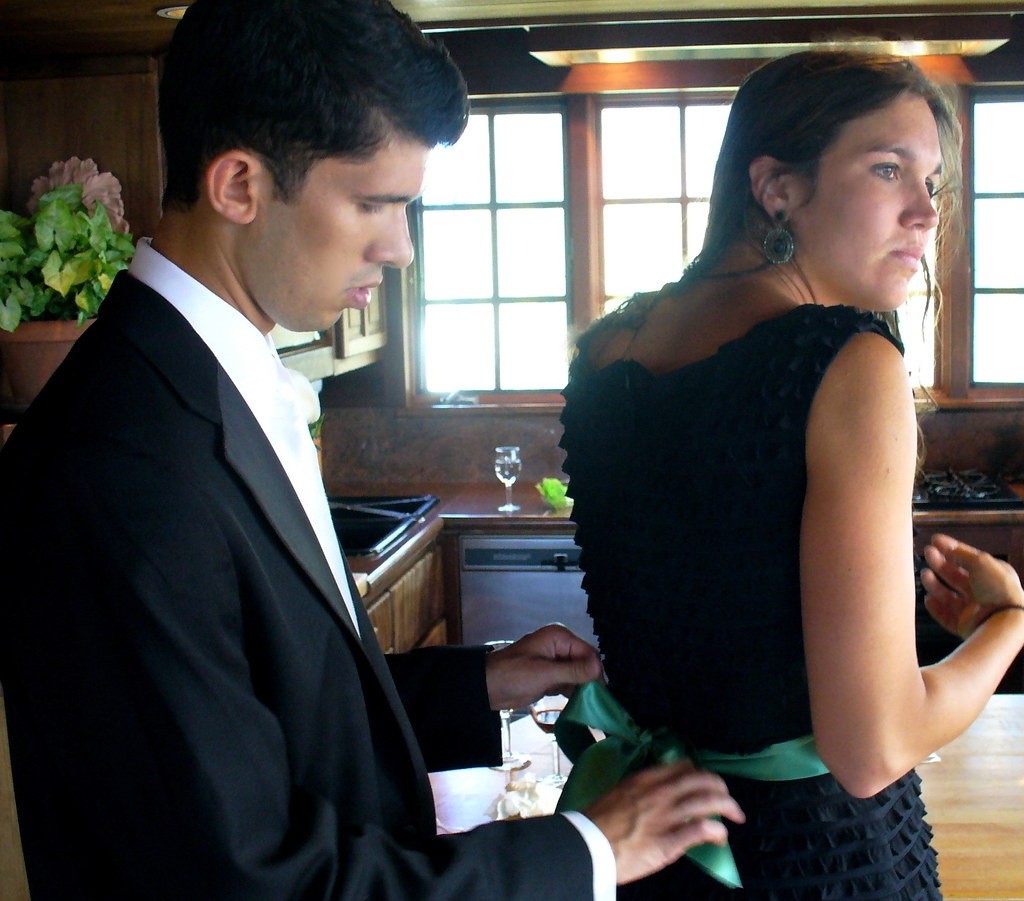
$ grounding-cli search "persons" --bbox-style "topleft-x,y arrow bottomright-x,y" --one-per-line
556,44 -> 1023,900
0,0 -> 747,901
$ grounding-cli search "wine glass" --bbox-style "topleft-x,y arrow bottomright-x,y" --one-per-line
494,446 -> 522,513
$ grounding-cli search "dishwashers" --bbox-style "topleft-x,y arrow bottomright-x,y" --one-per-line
460,536 -> 600,654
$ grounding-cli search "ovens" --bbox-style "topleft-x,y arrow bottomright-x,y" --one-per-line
910,527 -> 1024,694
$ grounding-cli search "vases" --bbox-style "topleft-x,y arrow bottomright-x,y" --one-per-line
0,318 -> 97,415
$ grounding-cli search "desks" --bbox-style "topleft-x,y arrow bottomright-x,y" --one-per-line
428,695 -> 1024,901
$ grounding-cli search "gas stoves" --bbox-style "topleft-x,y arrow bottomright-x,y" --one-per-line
907,464 -> 1024,511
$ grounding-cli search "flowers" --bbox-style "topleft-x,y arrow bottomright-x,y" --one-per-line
0,157 -> 135,333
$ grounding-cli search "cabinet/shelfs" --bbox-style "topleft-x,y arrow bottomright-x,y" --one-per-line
0,59 -> 388,381
367,545 -> 449,654
